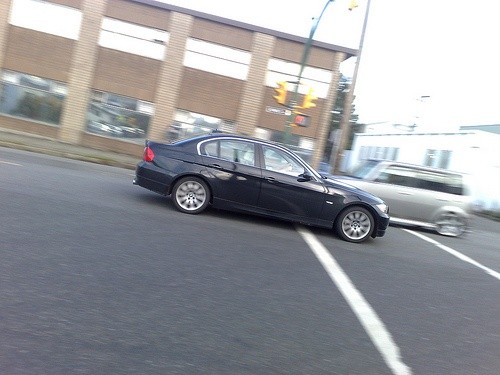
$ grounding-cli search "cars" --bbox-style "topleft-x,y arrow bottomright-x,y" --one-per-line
334,158 -> 470,237
132,133 -> 390,243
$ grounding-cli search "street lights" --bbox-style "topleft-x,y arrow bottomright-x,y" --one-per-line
272,0 -> 358,143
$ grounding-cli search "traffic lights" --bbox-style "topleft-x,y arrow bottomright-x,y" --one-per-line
273,80 -> 288,104
302,88 -> 317,108
